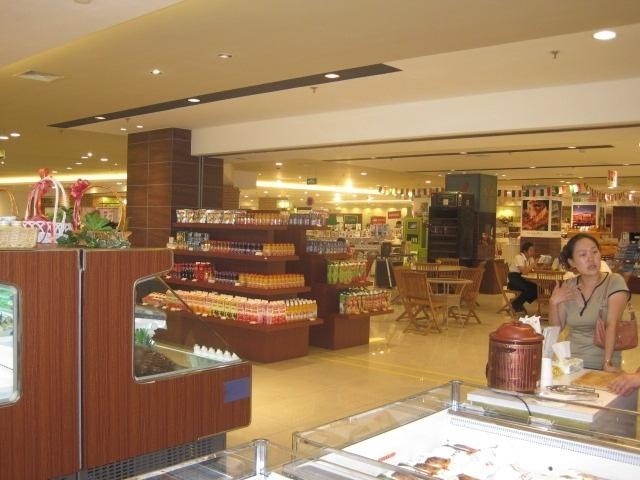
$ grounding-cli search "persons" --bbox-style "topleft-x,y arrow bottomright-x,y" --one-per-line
607,366 -> 639,398
506,241 -> 544,314
393,220 -> 402,228
547,232 -> 632,375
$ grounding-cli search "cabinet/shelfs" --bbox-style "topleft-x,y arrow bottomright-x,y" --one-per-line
141,221 -> 394,363
0,244 -> 252,480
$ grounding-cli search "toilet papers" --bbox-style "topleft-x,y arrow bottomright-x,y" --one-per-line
541,357 -> 553,389
553,340 -> 584,376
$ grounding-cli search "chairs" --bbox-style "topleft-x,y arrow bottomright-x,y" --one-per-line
390,257 -> 485,334
494,253 -> 634,323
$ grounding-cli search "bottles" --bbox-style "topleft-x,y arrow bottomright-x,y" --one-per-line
209,240 -> 296,259
176,264 -> 306,291
552,256 -> 560,272
339,288 -> 392,315
303,239 -> 348,254
234,207 -> 328,226
285,299 -> 318,323
325,261 -> 367,284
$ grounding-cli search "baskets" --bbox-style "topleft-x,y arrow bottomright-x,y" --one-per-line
71,182 -> 133,248
21,176 -> 73,245
0,186 -> 38,250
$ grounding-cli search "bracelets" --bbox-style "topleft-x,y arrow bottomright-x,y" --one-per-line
604,360 -> 613,366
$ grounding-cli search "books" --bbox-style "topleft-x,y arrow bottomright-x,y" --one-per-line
539,391 -> 600,402
546,384 -> 595,394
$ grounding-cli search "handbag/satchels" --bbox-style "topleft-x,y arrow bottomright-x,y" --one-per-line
592,274 -> 639,352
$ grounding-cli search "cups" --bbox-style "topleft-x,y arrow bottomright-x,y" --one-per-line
540,358 -> 554,390
558,265 -> 564,273
